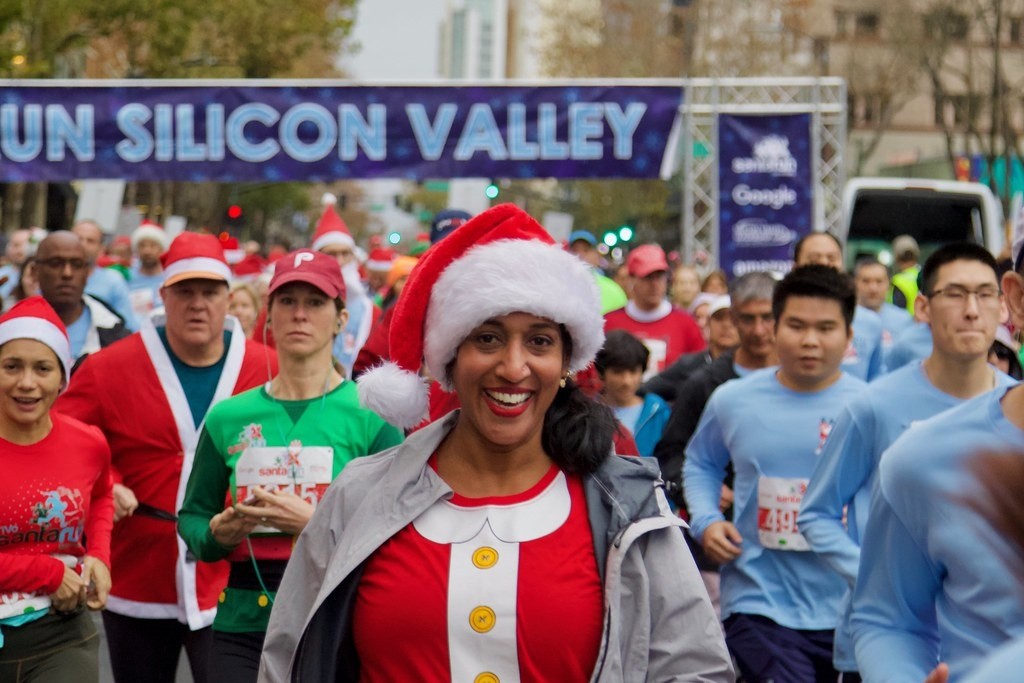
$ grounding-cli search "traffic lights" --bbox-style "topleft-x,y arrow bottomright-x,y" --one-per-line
218,197 -> 245,243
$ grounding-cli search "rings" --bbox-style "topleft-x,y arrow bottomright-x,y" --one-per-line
261,517 -> 267,522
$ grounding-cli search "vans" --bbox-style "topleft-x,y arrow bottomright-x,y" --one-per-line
835,176 -> 1011,267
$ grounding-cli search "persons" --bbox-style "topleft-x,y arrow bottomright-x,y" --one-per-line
0,202 -> 1024,683
257,202 -> 737,683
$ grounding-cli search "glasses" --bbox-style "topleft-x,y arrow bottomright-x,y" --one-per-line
925,284 -> 1004,310
33,256 -> 89,272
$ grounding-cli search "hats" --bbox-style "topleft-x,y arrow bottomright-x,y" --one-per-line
312,193 -> 355,258
365,246 -> 395,272
430,209 -> 472,247
158,233 -> 232,293
0,295 -> 71,397
270,247 -> 347,303
219,236 -> 286,280
895,235 -> 920,260
356,204 -> 607,427
708,296 -> 731,318
569,230 -> 598,250
164,272 -> 226,293
132,219 -> 168,252
627,245 -> 669,278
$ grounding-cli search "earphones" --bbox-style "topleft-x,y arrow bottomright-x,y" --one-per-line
266,317 -> 272,324
337,319 -> 341,329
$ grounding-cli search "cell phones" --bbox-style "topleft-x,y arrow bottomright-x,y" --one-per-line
239,485 -> 275,518
